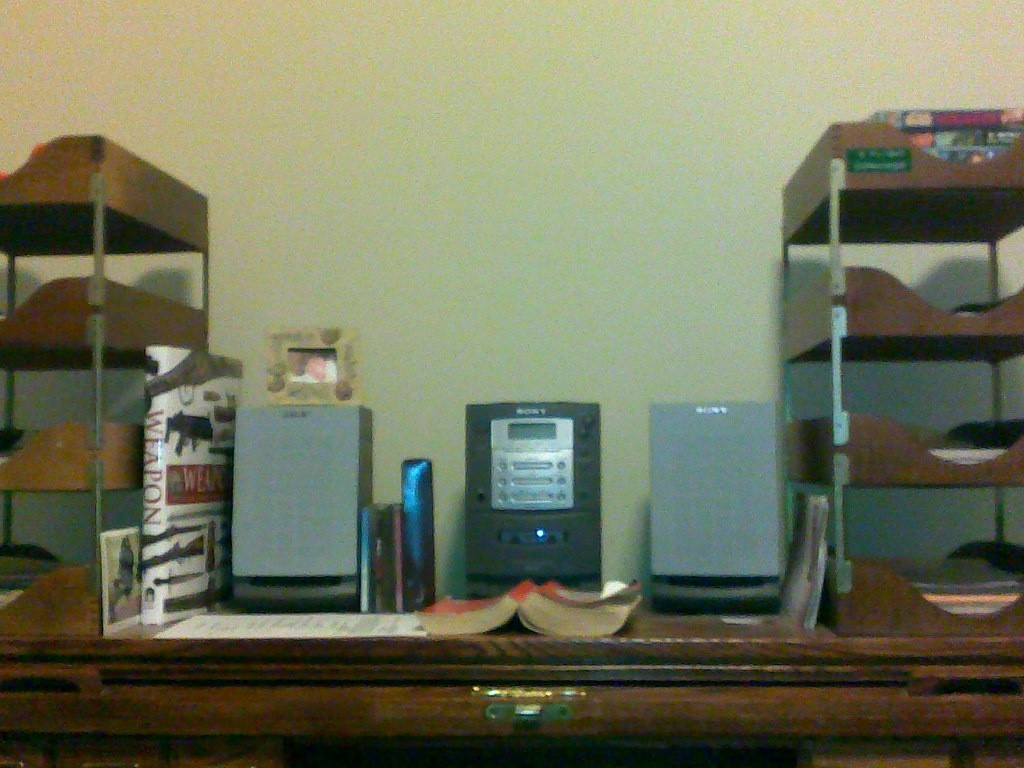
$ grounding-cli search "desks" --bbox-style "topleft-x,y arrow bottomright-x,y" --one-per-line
0,602 -> 1024,768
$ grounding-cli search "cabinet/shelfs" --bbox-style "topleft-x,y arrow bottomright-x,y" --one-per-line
784,113 -> 1024,637
1,133 -> 209,640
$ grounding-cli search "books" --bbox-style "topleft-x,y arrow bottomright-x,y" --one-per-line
869,109 -> 1024,165
929,419 -> 1024,465
361,503 -> 403,614
776,491 -> 830,630
887,556 -> 1023,616
415,579 -> 643,637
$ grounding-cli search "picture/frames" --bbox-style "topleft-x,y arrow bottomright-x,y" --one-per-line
265,327 -> 363,406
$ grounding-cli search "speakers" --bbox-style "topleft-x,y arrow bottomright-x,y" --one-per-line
231,405 -> 374,613
649,401 -> 783,615
